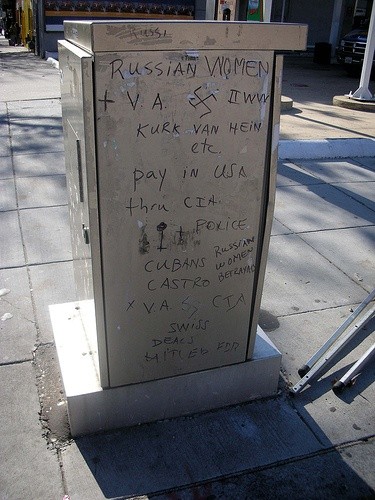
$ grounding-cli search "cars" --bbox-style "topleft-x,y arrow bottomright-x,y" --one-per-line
335,27 -> 369,77
